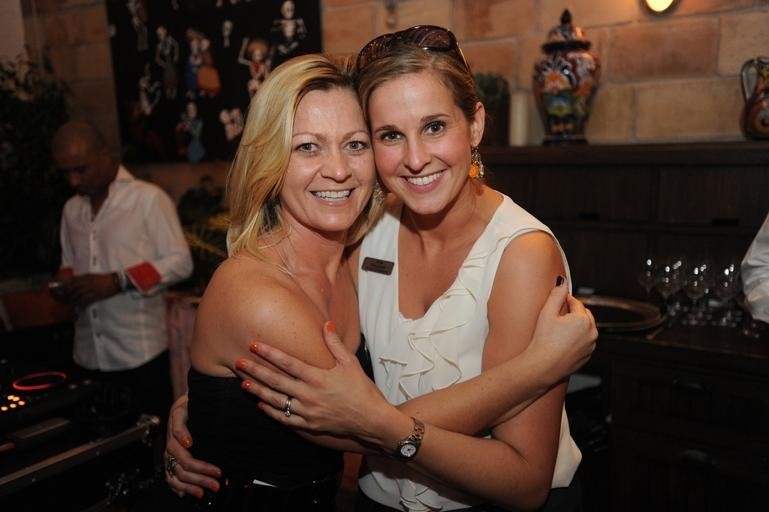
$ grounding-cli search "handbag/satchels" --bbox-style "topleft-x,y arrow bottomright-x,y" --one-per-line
199,66 -> 221,90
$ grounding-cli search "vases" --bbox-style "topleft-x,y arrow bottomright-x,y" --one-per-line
740,55 -> 769,140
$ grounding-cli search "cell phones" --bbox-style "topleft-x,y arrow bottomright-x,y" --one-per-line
49,281 -> 73,304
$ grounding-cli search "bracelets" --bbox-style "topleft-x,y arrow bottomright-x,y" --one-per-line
112,270 -> 127,292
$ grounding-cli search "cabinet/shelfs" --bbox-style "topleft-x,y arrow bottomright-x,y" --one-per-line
480,142 -> 769,317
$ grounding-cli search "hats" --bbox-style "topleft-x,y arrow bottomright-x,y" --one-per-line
248,42 -> 268,55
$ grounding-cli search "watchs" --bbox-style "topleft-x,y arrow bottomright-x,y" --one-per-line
394,414 -> 425,460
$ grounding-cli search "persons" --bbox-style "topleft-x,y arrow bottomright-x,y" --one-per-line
42,116 -> 193,420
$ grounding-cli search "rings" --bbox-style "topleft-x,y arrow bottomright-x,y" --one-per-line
167,455 -> 178,475
285,395 -> 293,416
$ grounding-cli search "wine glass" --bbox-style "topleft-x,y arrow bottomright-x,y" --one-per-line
635,255 -> 741,329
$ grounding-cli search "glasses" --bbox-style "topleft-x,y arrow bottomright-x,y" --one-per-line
353,24 -> 469,85
